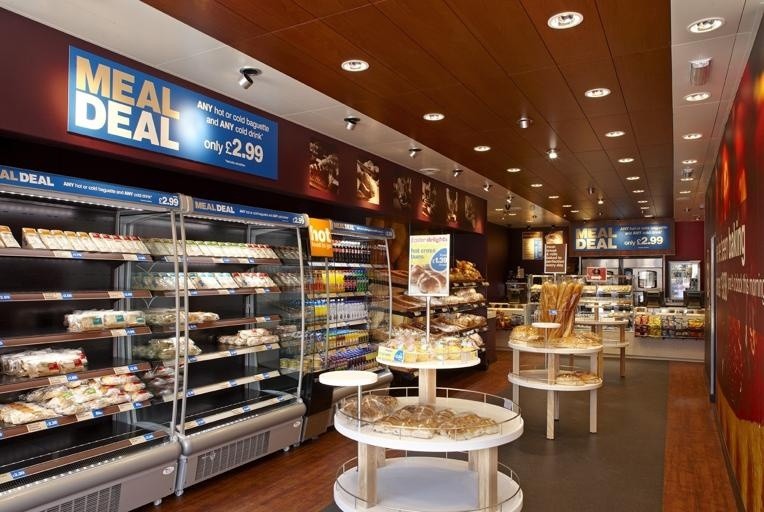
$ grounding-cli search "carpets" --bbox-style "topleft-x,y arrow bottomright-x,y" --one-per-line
319,355 -> 668,511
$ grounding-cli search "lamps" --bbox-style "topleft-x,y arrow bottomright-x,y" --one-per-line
408,147 -> 421,160
451,169 -> 462,178
344,116 -> 360,131
236,66 -> 262,91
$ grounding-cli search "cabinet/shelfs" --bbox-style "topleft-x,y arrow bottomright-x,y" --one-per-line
508,306 -> 604,440
181,196 -> 306,496
308,218 -> 391,440
2,165 -> 178,511
333,331 -> 525,512
368,278 -> 488,381
528,283 -> 633,378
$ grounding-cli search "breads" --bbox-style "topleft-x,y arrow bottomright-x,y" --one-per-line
510,281 -> 601,348
556,371 -> 600,385
339,329 -> 499,440
411,264 -> 446,293
439,260 -> 487,327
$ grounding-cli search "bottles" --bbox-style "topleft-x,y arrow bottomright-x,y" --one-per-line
289,239 -> 378,371
